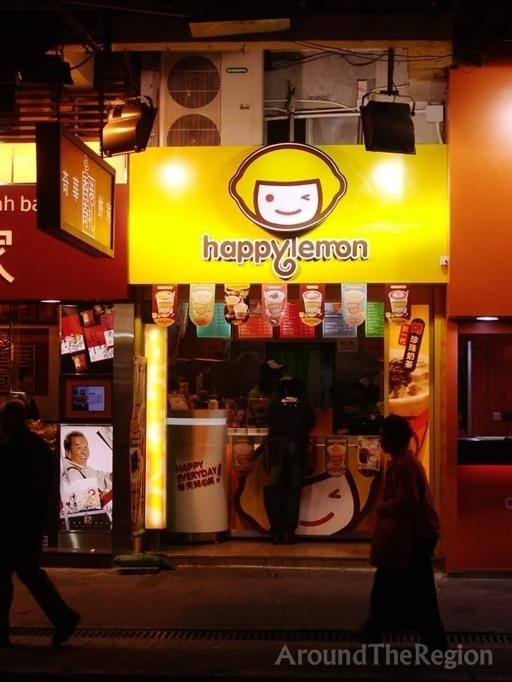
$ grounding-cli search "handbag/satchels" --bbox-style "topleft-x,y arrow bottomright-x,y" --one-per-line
369,515 -> 439,577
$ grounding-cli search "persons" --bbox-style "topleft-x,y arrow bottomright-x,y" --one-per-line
62,431 -> 113,527
247,359 -> 289,423
262,376 -> 316,545
0,400 -> 82,650
354,415 -> 449,660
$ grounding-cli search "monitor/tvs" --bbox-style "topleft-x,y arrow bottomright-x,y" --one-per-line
63,380 -> 112,419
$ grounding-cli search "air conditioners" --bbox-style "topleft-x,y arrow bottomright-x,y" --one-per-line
159,49 -> 266,147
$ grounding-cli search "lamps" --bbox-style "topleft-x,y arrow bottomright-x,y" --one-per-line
99,93 -> 160,159
358,48 -> 419,157
